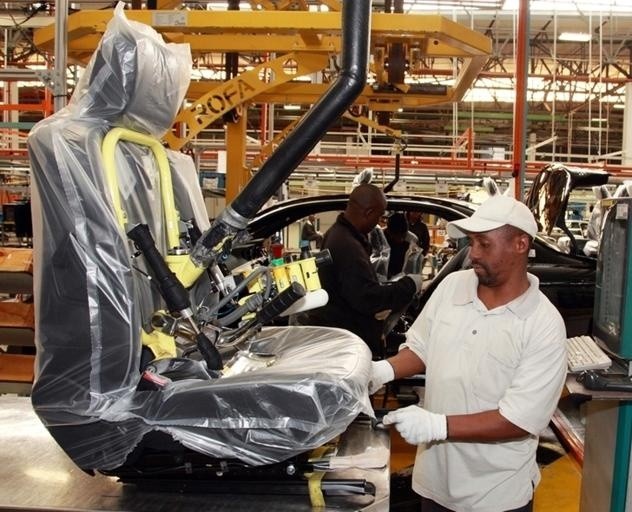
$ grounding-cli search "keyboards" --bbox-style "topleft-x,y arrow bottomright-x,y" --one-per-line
567,335 -> 613,371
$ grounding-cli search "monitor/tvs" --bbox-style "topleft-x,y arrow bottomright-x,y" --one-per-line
591,196 -> 632,377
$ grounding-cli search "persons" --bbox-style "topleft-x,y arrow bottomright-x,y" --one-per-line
301,215 -> 323,249
383,214 -> 425,278
377,209 -> 392,228
406,208 -> 428,253
366,192 -> 569,511
316,186 -> 421,358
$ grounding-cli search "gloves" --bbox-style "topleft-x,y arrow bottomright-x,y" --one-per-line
382,403 -> 448,448
408,272 -> 425,296
368,358 -> 396,396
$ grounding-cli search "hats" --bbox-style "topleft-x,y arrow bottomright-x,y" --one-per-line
445,194 -> 540,243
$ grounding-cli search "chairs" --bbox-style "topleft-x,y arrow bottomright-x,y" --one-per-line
25,22 -> 372,496
